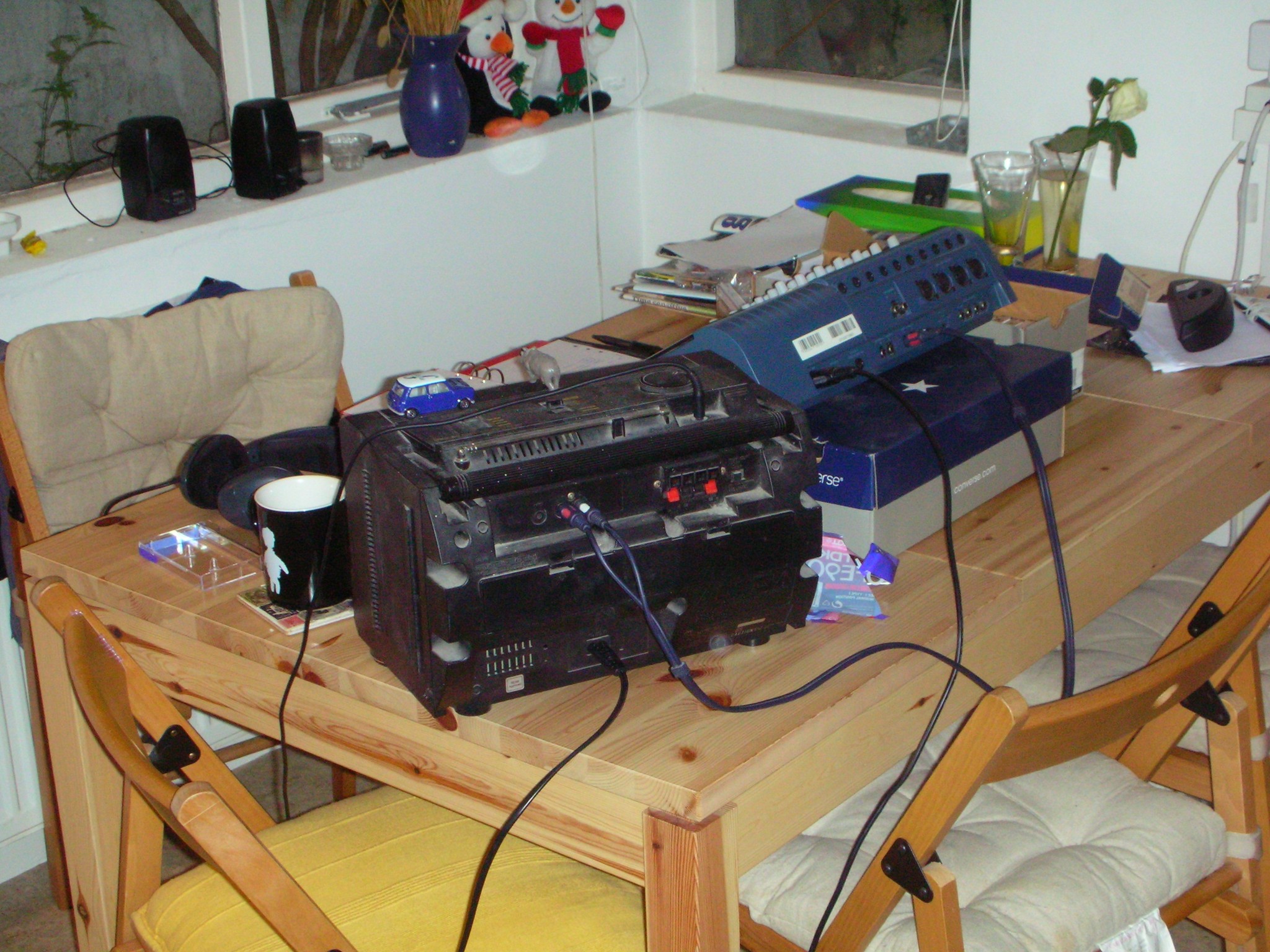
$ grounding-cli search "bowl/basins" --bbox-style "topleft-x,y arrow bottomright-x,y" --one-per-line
322,132 -> 372,172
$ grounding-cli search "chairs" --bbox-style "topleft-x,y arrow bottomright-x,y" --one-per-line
28,576 -> 647,952
0,269 -> 356,913
737,491 -> 1270,952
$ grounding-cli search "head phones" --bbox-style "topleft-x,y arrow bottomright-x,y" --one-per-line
176,425 -> 341,530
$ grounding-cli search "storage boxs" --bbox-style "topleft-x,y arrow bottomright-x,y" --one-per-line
138,519 -> 262,590
804,335 -> 1072,559
756,211 -> 1153,398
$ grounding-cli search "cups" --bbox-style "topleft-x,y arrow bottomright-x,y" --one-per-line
971,151 -> 1040,266
254,475 -> 350,610
299,131 -> 325,185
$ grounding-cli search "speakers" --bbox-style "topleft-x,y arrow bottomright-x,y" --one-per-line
230,96 -> 303,199
119,116 -> 195,223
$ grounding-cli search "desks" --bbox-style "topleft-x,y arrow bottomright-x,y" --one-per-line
18,256 -> 1270,952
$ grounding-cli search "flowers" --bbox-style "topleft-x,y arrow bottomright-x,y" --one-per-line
1046,74 -> 1147,264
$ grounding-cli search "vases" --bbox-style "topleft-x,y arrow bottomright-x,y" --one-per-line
396,26 -> 471,158
